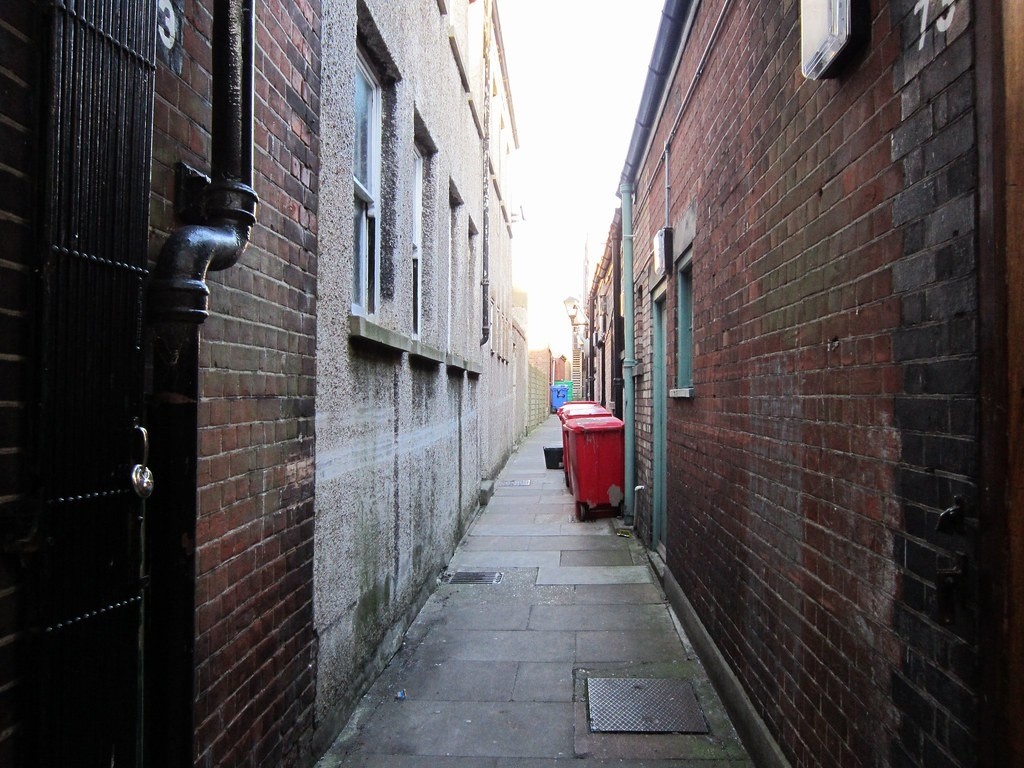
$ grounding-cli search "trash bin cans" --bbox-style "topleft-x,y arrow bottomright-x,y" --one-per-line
556,401 -> 626,522
549,384 -> 569,415
554,380 -> 572,400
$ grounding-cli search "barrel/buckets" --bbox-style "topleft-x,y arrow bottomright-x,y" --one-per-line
543,446 -> 565,470
